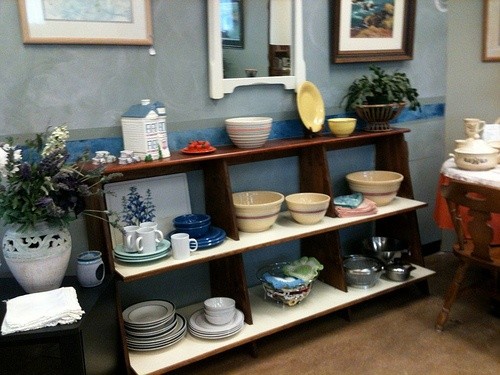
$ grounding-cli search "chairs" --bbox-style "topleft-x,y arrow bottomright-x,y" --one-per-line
432,175 -> 500,334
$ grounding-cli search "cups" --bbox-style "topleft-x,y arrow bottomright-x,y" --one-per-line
491,141 -> 500,164
245,68 -> 256,77
123,221 -> 198,260
464,118 -> 486,139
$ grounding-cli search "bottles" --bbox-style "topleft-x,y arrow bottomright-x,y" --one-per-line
76,250 -> 105,288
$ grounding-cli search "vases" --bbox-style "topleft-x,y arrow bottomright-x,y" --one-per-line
2,223 -> 72,293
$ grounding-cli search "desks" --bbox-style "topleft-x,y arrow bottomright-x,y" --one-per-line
431,157 -> 500,281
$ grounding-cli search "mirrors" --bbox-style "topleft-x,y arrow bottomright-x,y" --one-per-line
207,0 -> 305,100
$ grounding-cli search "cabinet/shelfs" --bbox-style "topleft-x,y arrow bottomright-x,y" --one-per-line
0,276 -> 102,375
73,126 -> 436,375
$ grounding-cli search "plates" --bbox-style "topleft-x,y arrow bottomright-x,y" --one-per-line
180,146 -> 217,153
188,308 -> 245,339
122,298 -> 187,351
114,226 -> 228,263
296,81 -> 326,132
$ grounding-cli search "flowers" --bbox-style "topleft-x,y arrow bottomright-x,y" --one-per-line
0,123 -> 128,235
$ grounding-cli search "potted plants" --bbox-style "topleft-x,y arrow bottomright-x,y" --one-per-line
337,67 -> 423,133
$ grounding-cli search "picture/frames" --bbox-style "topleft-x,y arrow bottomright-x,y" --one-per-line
481,0 -> 500,61
16,0 -> 154,45
332,0 -> 417,64
219,0 -> 245,49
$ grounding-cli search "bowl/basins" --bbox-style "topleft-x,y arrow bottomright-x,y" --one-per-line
232,190 -> 331,231
258,261 -> 313,306
328,117 -> 357,138
172,213 -> 212,237
448,134 -> 500,170
225,117 -> 274,148
346,170 -> 404,206
203,297 -> 236,326
344,236 -> 418,288
